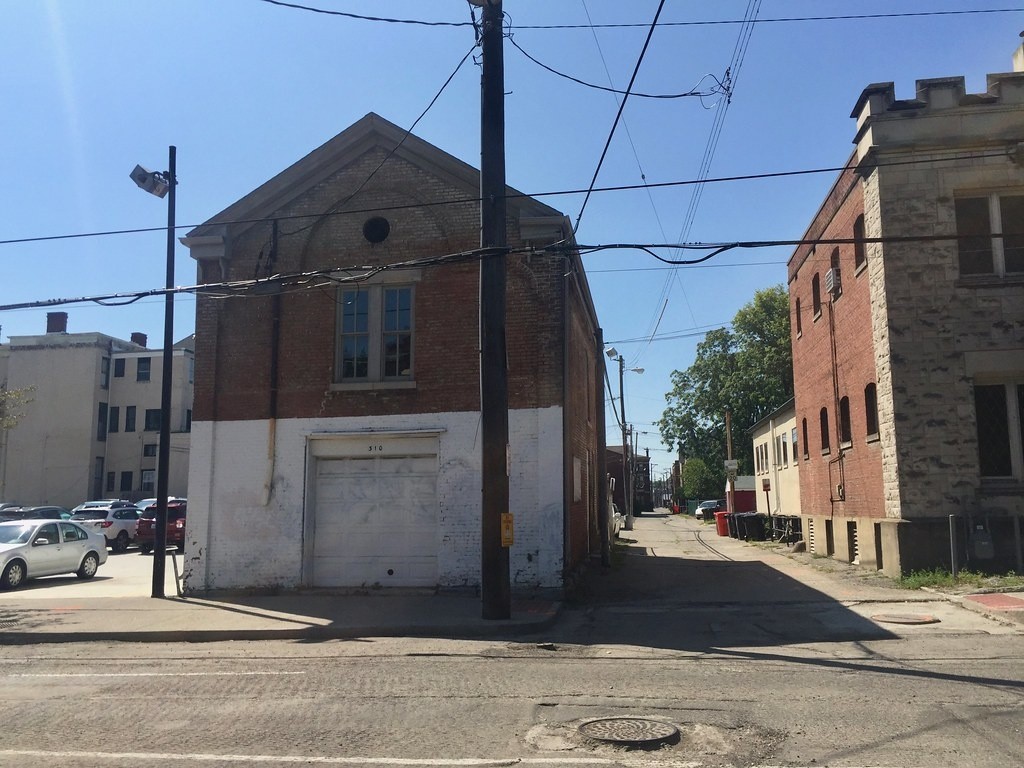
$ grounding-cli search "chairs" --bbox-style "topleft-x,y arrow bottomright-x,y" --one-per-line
64,532 -> 78,542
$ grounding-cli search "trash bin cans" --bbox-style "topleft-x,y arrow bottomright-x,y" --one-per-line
713,510 -> 767,543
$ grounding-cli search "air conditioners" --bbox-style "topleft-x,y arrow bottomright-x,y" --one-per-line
824,267 -> 840,293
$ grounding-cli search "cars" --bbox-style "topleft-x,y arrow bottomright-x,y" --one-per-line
611,503 -> 622,539
0,519 -> 107,592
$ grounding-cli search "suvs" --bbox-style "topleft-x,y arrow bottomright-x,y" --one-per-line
137,499 -> 187,554
0,502 -> 72,521
695,499 -> 727,520
66,504 -> 144,553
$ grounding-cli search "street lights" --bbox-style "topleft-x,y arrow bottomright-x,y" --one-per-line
129,165 -> 175,598
607,346 -> 644,531
